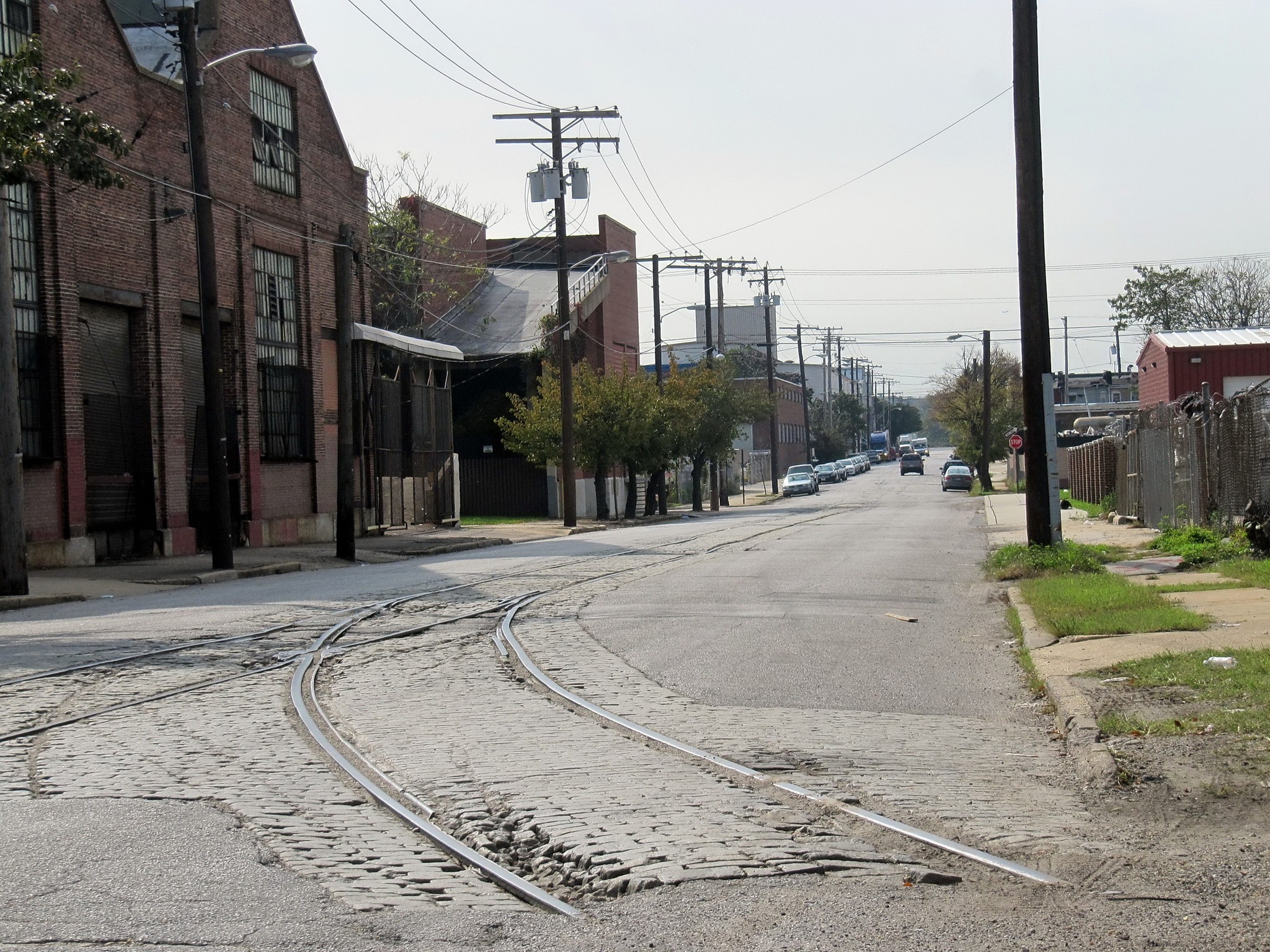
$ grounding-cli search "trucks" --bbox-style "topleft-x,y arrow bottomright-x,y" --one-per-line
898,434 -> 917,457
868,430 -> 897,462
911,437 -> 930,457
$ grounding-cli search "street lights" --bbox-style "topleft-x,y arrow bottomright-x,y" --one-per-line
946,329 -> 991,493
654,304 -> 708,515
183,43 -> 319,572
828,358 -> 850,429
821,363 -> 851,438
838,380 -> 859,392
766,334 -> 801,495
889,407 -> 902,443
799,353 -> 828,464
558,250 -> 632,526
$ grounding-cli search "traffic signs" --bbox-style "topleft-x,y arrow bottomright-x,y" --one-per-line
1003,424 -> 1020,439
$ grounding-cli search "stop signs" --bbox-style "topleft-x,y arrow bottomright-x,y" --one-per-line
1009,435 -> 1022,449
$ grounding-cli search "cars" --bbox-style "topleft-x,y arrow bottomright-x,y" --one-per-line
814,449 -> 881,484
942,466 -> 974,492
781,472 -> 814,498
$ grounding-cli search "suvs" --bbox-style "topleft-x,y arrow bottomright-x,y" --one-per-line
939,460 -> 967,485
899,453 -> 925,476
787,464 -> 820,491
